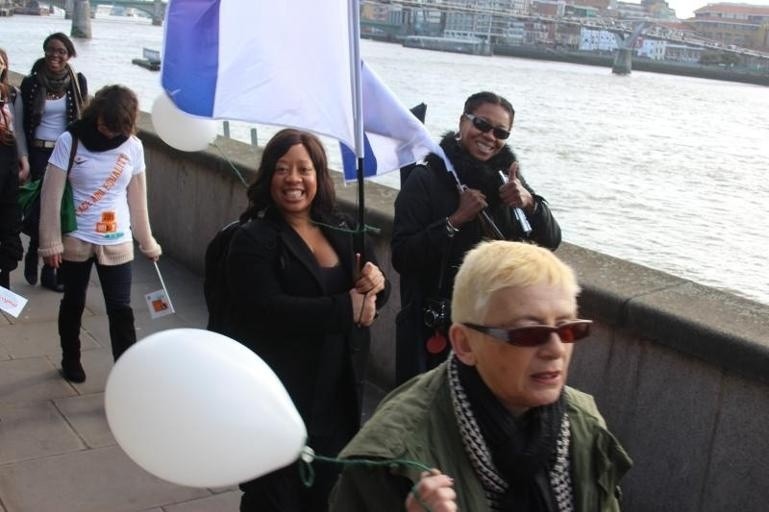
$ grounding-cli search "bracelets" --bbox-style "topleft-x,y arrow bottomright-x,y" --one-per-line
444,215 -> 461,238
532,199 -> 539,216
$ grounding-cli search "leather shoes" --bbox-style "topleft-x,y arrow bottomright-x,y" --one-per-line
40,263 -> 64,291
24,254 -> 38,286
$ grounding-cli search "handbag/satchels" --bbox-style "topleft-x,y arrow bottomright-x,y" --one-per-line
15,176 -> 78,236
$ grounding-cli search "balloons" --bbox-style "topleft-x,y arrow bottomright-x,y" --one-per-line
104,328 -> 316,491
151,86 -> 218,153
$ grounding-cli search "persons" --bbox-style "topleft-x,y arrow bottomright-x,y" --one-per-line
327,239 -> 635,512
0,83 -> 24,291
36,84 -> 165,383
19,32 -> 88,293
0,47 -> 32,182
215,127 -> 393,511
389,90 -> 562,388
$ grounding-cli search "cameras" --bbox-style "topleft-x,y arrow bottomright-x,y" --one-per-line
415,294 -> 446,330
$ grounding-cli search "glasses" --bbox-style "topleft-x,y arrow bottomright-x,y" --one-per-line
464,112 -> 511,140
458,318 -> 594,347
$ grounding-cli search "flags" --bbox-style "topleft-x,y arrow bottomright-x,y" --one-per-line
340,59 -> 453,185
149,0 -> 366,161
